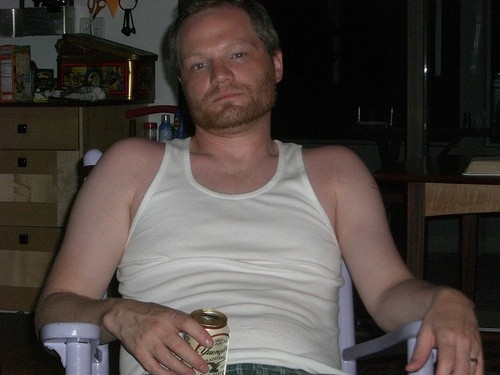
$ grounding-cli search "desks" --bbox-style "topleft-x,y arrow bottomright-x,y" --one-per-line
371,170 -> 500,346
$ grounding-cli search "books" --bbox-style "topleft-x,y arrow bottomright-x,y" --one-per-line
460,156 -> 500,176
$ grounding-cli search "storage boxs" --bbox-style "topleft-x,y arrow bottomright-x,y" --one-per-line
0,6 -> 75,38
0,45 -> 33,102
55,32 -> 159,101
32,77 -> 57,97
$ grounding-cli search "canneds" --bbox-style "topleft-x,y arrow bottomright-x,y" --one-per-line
182,308 -> 230,375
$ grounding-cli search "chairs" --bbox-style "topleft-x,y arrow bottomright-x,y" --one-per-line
39,147 -> 439,375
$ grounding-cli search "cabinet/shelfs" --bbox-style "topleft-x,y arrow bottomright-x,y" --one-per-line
0,98 -> 154,315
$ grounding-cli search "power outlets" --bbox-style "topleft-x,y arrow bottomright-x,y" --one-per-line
80,17 -> 105,39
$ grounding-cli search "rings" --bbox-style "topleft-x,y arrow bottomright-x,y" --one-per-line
470,357 -> 478,364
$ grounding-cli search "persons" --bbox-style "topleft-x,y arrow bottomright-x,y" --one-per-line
34,0 -> 484,375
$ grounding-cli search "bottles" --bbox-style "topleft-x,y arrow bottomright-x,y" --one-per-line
143,122 -> 157,141
159,114 -> 173,143
